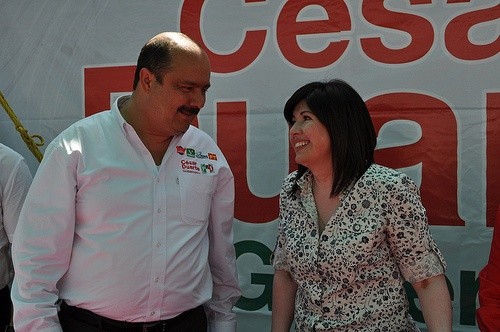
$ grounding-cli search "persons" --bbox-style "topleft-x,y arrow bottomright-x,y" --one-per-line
272,78 -> 454,332
0,144 -> 36,331
10,32 -> 242,332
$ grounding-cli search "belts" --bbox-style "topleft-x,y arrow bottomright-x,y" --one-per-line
56,299 -> 204,332
